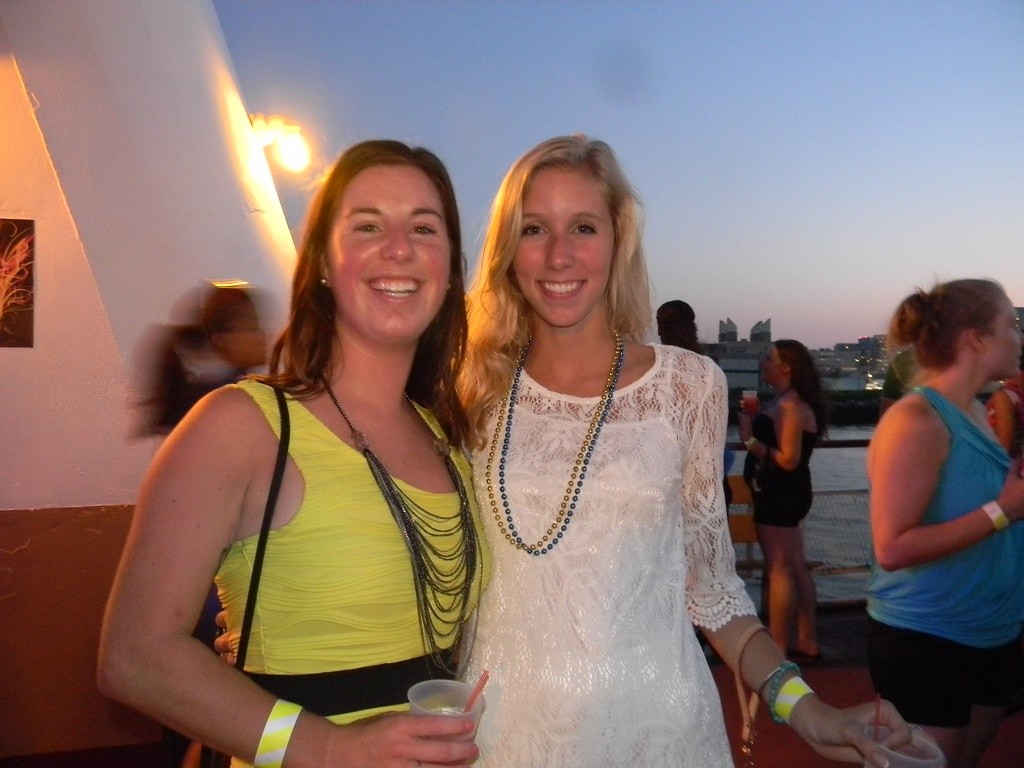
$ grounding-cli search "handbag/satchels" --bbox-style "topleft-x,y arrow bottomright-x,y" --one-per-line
181,740 -> 231,768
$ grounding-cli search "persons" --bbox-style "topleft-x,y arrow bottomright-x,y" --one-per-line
738,339 -> 824,668
657,298 -> 733,517
215,133 -> 947,768
864,279 -> 1024,768
99,137 -> 492,768
136,281 -> 268,654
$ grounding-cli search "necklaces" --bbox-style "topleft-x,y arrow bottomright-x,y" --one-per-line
773,385 -> 791,404
318,373 -> 483,682
486,327 -> 623,556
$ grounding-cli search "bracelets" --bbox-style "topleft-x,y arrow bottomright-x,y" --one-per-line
758,661 -> 816,724
251,699 -> 303,767
745,437 -> 756,449
982,501 -> 1008,531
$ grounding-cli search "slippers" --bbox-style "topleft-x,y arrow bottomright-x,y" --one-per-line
786,649 -> 824,668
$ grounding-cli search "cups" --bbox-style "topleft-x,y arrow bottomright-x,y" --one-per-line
407,679 -> 484,765
742,390 -> 758,413
862,726 -> 944,768
